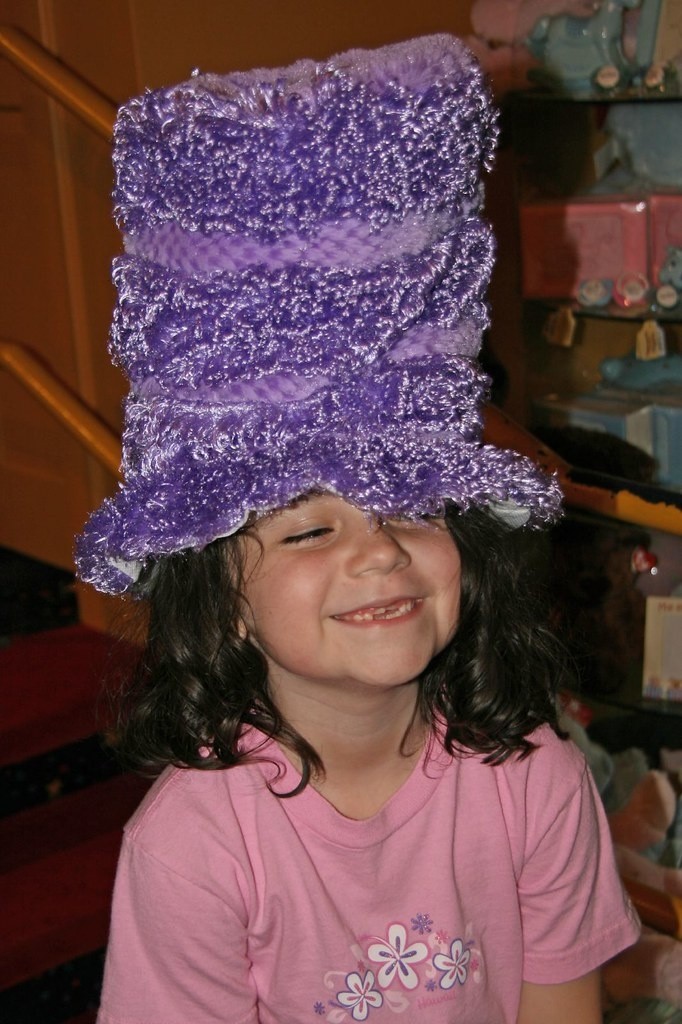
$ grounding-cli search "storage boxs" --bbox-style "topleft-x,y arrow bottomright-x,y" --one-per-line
521,194 -> 682,708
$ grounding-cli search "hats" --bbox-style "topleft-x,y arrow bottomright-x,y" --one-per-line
72,32 -> 564,594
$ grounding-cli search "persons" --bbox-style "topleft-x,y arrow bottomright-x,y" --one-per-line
92,34 -> 605,1024
